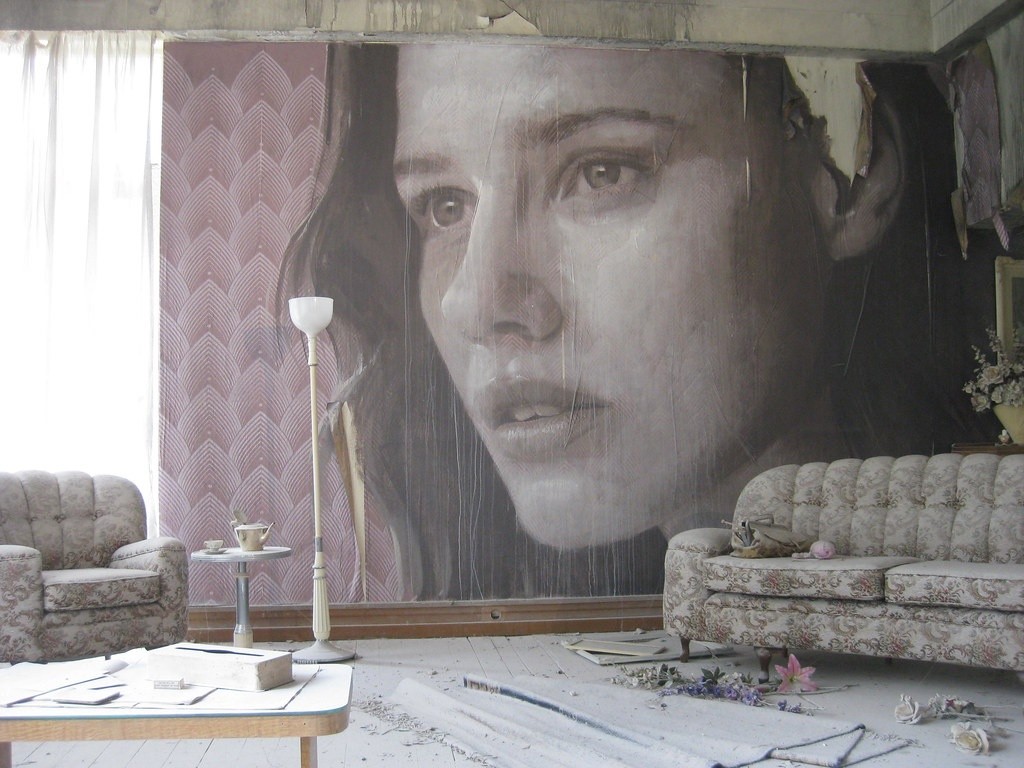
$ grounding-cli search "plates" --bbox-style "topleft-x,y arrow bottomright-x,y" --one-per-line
200,549 -> 227,554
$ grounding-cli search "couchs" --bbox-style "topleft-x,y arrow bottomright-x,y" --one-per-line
661,453 -> 1024,688
0,469 -> 189,665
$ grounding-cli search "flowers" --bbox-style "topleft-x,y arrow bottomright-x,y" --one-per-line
613,649 -> 822,718
962,323 -> 1024,412
892,692 -> 1015,756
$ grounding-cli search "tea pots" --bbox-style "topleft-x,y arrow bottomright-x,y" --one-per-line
233,519 -> 275,550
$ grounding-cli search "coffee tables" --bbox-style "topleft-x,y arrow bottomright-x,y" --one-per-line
189,545 -> 292,650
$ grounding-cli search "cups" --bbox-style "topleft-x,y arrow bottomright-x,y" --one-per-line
204,540 -> 223,551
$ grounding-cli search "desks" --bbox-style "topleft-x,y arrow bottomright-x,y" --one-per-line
1,664 -> 354,768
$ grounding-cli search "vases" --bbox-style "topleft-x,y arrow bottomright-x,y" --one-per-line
992,404 -> 1024,446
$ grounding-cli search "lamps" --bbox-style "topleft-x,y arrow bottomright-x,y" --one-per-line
288,295 -> 358,663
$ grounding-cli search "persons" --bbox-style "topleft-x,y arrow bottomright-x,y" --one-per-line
272,40 -> 956,599
